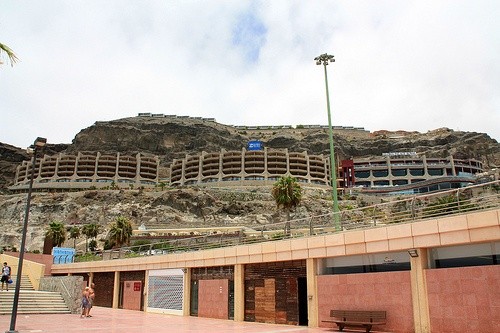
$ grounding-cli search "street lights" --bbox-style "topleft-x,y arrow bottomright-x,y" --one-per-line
314,52 -> 342,231
6,136 -> 49,333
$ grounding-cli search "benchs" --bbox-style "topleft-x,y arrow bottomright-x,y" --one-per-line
321,309 -> 386,333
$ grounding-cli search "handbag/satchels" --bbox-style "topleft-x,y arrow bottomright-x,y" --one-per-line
7,278 -> 12,284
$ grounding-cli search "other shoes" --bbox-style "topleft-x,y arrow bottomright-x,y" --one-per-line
81,315 -> 92,318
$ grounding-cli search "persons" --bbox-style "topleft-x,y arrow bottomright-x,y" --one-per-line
79,287 -> 92,319
85,282 -> 95,318
0,262 -> 13,291
